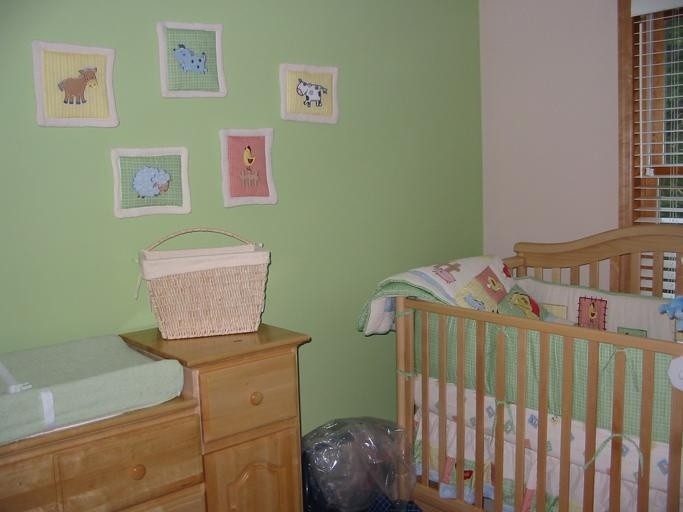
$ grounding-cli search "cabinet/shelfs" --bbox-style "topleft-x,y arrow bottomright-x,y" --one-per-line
119,322 -> 312,512
1,395 -> 208,512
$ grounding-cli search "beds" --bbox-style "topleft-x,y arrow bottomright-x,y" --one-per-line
392,223 -> 683,512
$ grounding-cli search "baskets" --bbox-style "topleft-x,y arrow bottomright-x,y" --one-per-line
140,228 -> 271,340
